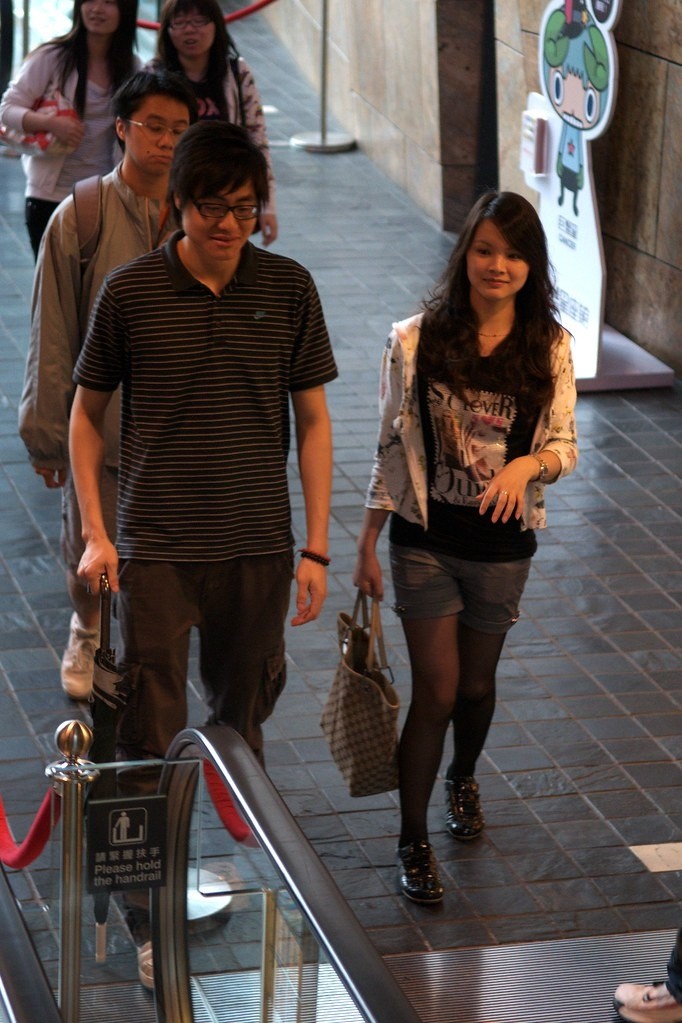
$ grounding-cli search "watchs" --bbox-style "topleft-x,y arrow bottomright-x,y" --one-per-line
527,454 -> 548,483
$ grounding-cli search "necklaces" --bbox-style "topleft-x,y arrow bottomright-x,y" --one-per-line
478,331 -> 508,338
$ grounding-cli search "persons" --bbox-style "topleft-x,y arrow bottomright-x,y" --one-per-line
142,0 -> 278,247
0,0 -> 142,265
352,189 -> 579,904
13,70 -> 199,699
68,118 -> 340,989
613,925 -> 682,1023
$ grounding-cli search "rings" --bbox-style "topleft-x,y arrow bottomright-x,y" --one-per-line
500,491 -> 508,495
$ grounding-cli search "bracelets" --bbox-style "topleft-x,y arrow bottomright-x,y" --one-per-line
294,548 -> 331,566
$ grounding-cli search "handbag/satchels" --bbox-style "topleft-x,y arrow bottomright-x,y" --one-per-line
0,38 -> 80,157
319,589 -> 402,796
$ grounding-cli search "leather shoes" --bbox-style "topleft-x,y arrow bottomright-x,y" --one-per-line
444,764 -> 484,839
395,840 -> 444,904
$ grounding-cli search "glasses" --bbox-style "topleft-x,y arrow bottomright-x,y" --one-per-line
190,191 -> 261,221
169,14 -> 211,30
126,118 -> 189,143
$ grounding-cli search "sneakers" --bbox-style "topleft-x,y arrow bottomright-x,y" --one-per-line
60,611 -> 100,698
137,941 -> 154,991
613,982 -> 682,1023
